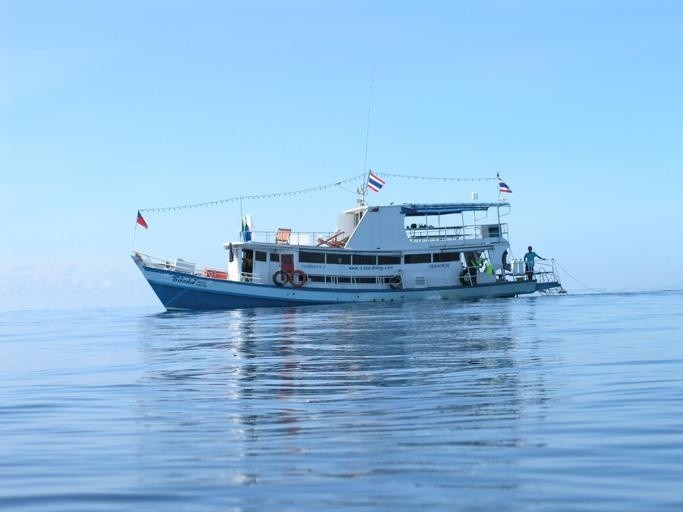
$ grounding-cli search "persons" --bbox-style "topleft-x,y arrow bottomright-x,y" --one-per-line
523,246 -> 547,280
470,251 -> 484,285
245,253 -> 253,281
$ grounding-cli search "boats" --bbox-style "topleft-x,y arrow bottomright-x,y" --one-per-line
129,167 -> 570,316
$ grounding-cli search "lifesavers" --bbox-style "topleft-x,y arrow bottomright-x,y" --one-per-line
459,269 -> 477,286
272,269 -> 307,287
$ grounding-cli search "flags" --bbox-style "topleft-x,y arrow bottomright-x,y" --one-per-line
367,173 -> 385,192
137,211 -> 148,229
497,177 -> 512,193
